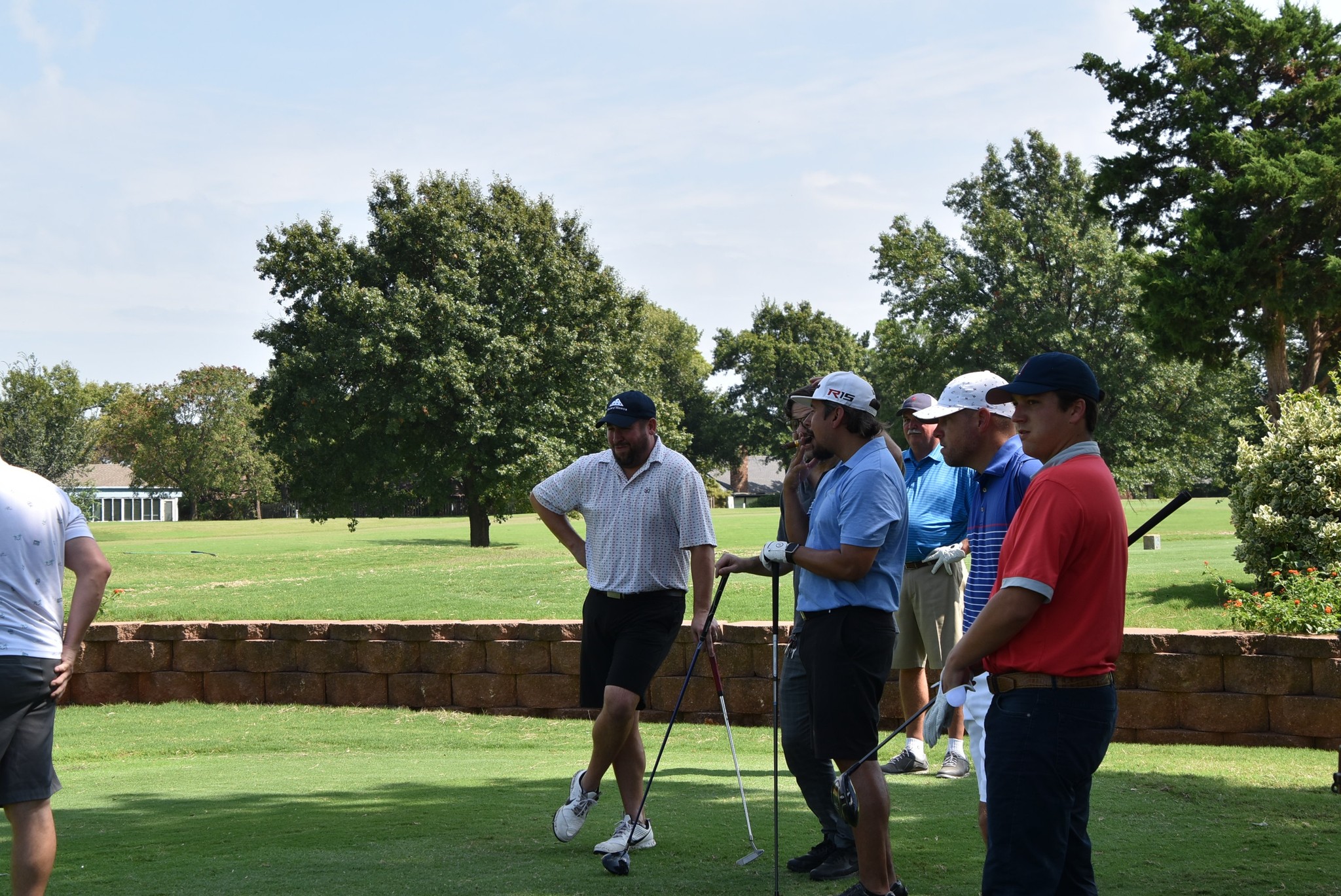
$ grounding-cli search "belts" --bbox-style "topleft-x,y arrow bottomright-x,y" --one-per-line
798,606 -> 895,621
986,671 -> 1114,695
904,558 -> 940,570
589,587 -> 688,599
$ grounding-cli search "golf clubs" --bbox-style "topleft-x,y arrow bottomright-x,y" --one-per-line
829,491 -> 1193,826
770,562 -> 781,896
602,563 -> 731,876
705,634 -> 765,866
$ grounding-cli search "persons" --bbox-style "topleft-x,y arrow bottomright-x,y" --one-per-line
922,351 -> 1129,896
714,371 -> 908,896
529,390 -> 724,856
0,456 -> 112,896
880,370 -> 1045,846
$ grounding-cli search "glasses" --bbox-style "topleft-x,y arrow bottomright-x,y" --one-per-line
784,416 -> 811,434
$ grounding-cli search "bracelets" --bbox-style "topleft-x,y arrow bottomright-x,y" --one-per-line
784,543 -> 801,564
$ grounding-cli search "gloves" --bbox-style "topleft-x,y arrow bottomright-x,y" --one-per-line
922,665 -> 976,749
921,541 -> 966,576
759,540 -> 788,572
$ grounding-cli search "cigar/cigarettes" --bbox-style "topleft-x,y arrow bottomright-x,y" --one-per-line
777,436 -> 812,450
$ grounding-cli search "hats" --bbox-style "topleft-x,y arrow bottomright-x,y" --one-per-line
595,389 -> 657,428
912,370 -> 1016,420
790,371 -> 878,417
895,393 -> 938,417
985,351 -> 1100,405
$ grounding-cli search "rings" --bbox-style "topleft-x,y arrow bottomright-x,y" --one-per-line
713,634 -> 717,638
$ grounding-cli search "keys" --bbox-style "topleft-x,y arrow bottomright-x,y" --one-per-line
783,633 -> 797,659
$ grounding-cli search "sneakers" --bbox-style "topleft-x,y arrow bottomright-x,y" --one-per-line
837,881 -> 896,896
786,839 -> 846,872
552,768 -> 602,843
890,879 -> 908,896
879,745 -> 929,775
936,744 -> 970,779
809,848 -> 859,879
593,811 -> 657,854
